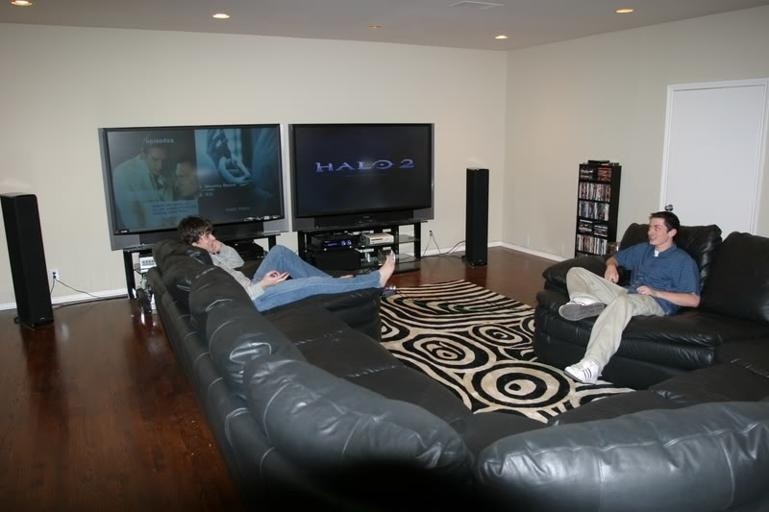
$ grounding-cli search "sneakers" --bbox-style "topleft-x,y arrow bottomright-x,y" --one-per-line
565,359 -> 599,384
559,297 -> 604,322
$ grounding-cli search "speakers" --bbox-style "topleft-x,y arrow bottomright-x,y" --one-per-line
1,193 -> 53,328
466,164 -> 490,269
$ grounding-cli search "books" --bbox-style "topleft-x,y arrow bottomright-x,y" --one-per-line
576,159 -> 613,258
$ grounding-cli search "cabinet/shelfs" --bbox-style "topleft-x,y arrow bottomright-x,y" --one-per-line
574,160 -> 621,258
124,232 -> 282,300
299,222 -> 421,278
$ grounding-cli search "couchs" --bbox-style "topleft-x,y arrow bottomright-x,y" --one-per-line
140,223 -> 769,512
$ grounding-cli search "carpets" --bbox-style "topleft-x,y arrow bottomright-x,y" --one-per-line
380,277 -> 638,424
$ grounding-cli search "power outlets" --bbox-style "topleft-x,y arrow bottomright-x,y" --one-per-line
427,229 -> 434,238
50,270 -> 60,281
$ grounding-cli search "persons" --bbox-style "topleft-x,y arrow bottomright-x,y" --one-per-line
558,211 -> 703,384
178,214 -> 397,313
113,129 -> 188,232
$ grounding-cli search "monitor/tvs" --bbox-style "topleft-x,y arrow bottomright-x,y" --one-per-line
292,121 -> 435,233
98,123 -> 288,253
